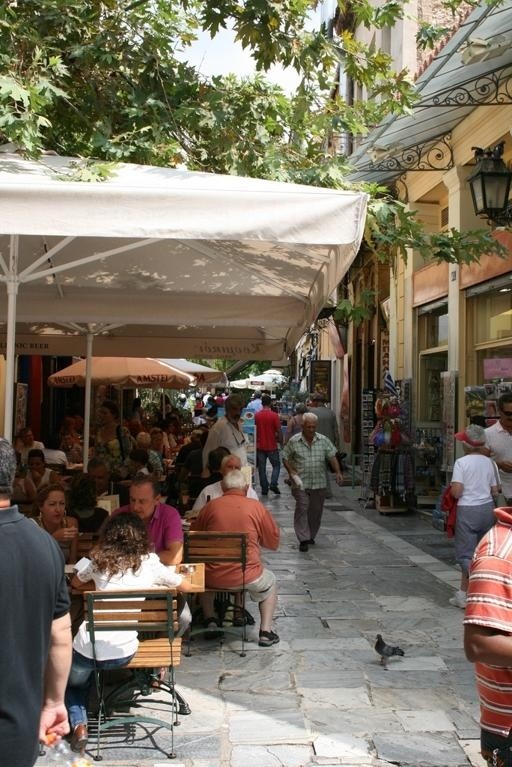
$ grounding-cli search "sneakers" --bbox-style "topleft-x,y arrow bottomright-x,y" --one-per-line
261,489 -> 269,495
454,430 -> 486,447
269,483 -> 281,495
308,538 -> 315,544
448,591 -> 468,609
299,540 -> 309,552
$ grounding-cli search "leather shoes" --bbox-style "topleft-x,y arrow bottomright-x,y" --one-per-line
70,724 -> 89,753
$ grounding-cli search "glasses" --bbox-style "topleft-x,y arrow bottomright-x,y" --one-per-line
232,431 -> 249,449
501,409 -> 512,417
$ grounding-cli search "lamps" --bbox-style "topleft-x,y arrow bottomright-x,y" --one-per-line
466,140 -> 508,225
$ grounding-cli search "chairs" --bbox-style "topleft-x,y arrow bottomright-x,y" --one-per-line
183,530 -> 248,654
71,535 -> 98,564
82,587 -> 183,761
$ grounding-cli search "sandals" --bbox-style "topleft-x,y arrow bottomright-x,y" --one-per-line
258,630 -> 280,646
204,616 -> 223,639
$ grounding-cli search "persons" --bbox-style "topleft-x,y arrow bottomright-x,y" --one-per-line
461,506 -> 512,767
309,392 -> 340,500
280,413 -> 344,552
223,392 -> 230,401
202,395 -> 248,479
254,395 -> 281,496
60,431 -> 84,469
215,394 -> 224,406
65,511 -> 192,753
0,435 -> 73,767
87,457 -> 129,513
110,475 -> 192,692
65,473 -> 109,533
15,427 -> 45,474
42,432 -> 68,467
189,469 -> 280,647
247,390 -> 264,413
94,400 -> 131,475
202,391 -> 211,407
205,397 -> 217,417
185,455 -> 259,626
131,392 -> 201,473
23,448 -> 61,496
448,424 -> 502,608
284,401 -> 309,487
484,393 -> 512,507
28,482 -> 79,564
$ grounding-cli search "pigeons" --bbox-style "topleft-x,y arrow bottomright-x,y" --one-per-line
374,632 -> 405,671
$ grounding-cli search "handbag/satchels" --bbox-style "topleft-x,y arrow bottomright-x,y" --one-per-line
490,457 -> 508,507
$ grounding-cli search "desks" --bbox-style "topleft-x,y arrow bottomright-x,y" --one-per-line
61,563 -> 206,715
